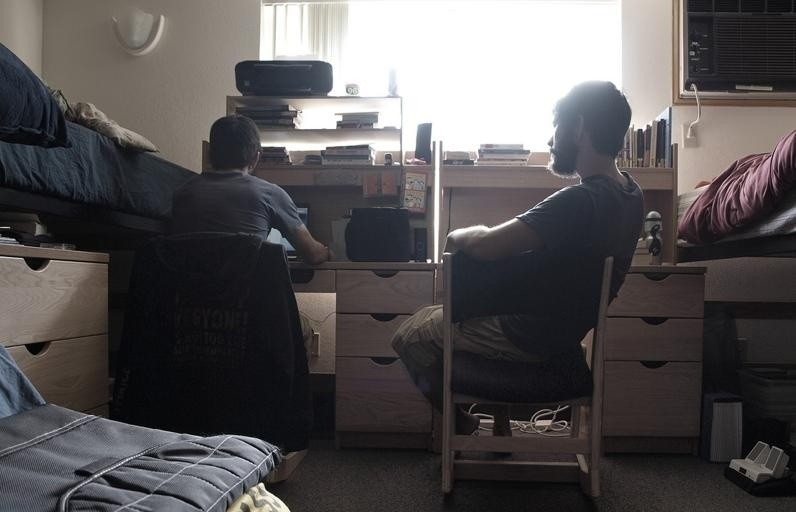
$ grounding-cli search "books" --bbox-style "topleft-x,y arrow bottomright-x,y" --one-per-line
238,104 -> 386,168
472,114 -> 669,172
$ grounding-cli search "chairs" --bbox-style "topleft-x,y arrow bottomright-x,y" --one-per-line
136,233 -> 268,432
441,249 -> 615,500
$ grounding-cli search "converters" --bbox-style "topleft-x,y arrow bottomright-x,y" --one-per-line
535,420 -> 552,432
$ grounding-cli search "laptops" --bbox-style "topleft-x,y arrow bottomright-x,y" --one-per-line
267,202 -> 310,261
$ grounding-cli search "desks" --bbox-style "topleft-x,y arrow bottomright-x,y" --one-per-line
436,142 -> 708,457
203,94 -> 437,450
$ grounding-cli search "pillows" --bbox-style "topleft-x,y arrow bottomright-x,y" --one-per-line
0,43 -> 73,148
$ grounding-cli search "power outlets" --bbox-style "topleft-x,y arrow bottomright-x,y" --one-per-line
681,124 -> 697,148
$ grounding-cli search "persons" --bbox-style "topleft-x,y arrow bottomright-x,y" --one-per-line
137,116 -> 340,441
384,73 -> 649,444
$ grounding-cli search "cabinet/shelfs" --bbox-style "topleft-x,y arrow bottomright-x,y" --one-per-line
0,246 -> 110,420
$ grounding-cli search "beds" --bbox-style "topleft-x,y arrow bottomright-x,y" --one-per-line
0,117 -> 199,250
677,130 -> 796,395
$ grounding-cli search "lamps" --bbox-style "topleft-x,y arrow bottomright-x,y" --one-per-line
109,6 -> 166,57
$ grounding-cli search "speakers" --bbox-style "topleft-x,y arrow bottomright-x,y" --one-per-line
235,61 -> 333,96
351,207 -> 410,262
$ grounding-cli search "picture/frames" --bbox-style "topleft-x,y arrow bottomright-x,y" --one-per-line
671,0 -> 796,108
